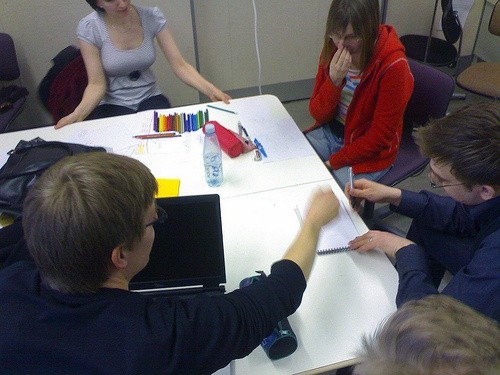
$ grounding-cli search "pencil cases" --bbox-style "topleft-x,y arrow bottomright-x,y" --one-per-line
202,120 -> 243,159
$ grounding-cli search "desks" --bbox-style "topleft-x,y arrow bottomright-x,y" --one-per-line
0,93 -> 399,375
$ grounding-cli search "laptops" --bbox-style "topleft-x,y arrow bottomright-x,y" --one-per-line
127,195 -> 235,375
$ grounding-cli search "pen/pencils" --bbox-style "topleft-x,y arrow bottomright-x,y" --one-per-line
132,133 -> 182,139
153,108 -> 209,134
253,138 -> 268,159
207,104 -> 235,114
348,167 -> 353,204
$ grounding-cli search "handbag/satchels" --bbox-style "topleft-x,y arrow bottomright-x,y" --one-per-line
0,136 -> 106,217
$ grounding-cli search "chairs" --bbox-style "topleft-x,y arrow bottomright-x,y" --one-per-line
456,0 -> 500,103
359,55 -> 454,239
398,0 -> 466,100
35,43 -> 96,123
0,33 -> 29,135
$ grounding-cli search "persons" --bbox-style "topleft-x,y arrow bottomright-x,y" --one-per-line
344,99 -> 500,327
0,150 -> 342,375
55,0 -> 233,129
352,293 -> 500,375
301,0 -> 414,207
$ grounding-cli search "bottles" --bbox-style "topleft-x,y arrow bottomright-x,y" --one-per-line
202,123 -> 224,188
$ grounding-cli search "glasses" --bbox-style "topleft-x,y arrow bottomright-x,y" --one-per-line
326,33 -> 361,41
142,206 -> 168,230
427,171 -> 467,188
